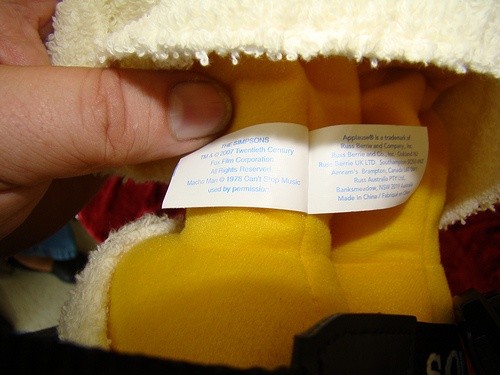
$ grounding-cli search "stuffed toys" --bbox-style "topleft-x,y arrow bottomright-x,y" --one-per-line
42,1 -> 499,374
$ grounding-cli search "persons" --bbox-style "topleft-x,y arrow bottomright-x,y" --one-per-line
0,0 -> 233,257
